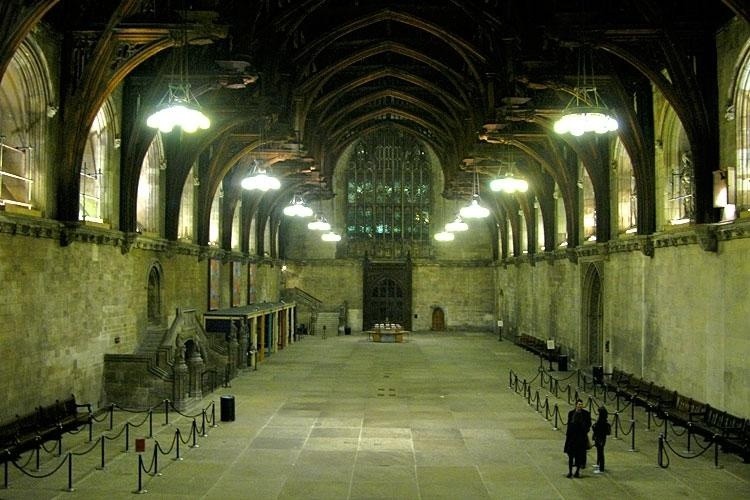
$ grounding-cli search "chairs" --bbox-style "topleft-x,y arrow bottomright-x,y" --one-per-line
0,393 -> 93,465
514,333 -> 560,362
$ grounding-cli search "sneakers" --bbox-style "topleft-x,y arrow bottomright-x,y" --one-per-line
592,464 -> 605,473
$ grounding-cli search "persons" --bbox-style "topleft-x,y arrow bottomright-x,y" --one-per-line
592,406 -> 611,474
563,399 -> 591,478
230,317 -> 249,338
339,300 -> 348,320
175,333 -> 186,358
247,341 -> 255,367
195,330 -> 201,353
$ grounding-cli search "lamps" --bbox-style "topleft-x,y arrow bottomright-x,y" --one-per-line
283,149 -> 314,218
433,38 -> 619,242
146,28 -> 215,135
320,231 -> 343,242
306,177 -> 332,232
243,122 -> 281,194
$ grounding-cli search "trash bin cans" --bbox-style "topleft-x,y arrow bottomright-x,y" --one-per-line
220,395 -> 235,422
592,366 -> 603,388
345,326 -> 351,335
558,355 -> 567,371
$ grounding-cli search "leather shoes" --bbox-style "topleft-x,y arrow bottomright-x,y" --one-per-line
566,472 -> 579,478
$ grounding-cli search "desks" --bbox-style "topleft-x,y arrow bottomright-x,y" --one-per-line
366,330 -> 410,343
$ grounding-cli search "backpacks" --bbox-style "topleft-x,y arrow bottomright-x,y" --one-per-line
605,422 -> 611,435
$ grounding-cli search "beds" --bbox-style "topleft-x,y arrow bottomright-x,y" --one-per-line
599,367 -> 750,464
